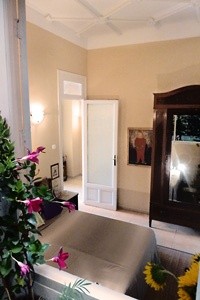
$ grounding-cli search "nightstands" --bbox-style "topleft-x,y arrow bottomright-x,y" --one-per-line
56,190 -> 78,211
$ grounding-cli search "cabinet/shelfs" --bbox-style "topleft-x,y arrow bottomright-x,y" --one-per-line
148,84 -> 200,234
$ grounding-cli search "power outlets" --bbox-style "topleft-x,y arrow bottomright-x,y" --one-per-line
52,145 -> 56,149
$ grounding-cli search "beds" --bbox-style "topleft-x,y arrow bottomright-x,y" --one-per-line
13,179 -> 157,299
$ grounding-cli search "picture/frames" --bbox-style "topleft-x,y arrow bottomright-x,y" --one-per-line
50,162 -> 59,180
126,127 -> 153,167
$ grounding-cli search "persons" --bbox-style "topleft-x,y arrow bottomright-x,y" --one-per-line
134,131 -> 147,164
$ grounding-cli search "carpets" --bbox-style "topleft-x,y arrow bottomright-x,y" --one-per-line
136,244 -> 194,299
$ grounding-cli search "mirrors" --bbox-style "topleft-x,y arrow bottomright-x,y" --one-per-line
168,141 -> 200,206
